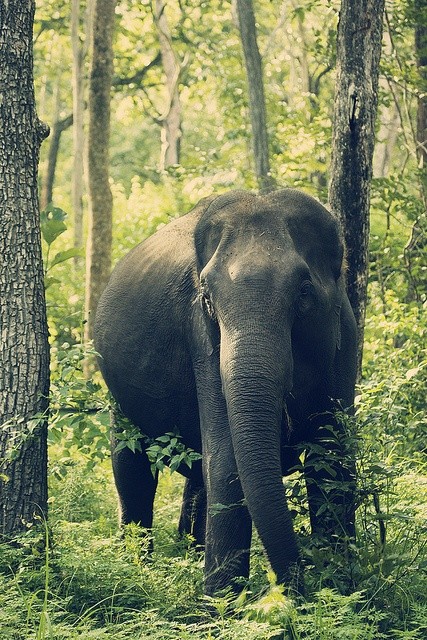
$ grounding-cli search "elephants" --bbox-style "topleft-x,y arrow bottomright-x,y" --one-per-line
94,190 -> 359,611
